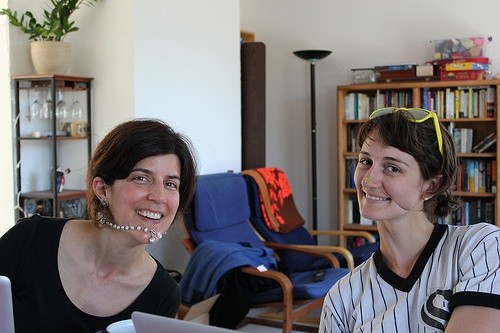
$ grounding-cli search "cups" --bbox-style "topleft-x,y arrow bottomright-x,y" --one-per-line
71,120 -> 86,137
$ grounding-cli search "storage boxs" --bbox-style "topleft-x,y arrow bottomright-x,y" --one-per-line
438,57 -> 492,80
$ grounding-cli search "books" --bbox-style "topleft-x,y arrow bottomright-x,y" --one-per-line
338,88 -> 497,224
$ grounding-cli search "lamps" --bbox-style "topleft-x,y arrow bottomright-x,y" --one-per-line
292,49 -> 333,246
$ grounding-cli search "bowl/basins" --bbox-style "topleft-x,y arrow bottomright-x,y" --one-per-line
106,319 -> 138,333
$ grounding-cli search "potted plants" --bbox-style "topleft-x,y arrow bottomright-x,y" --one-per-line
0,0 -> 98,76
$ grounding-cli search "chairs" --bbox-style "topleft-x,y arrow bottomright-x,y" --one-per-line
176,170 -> 354,333
242,167 -> 378,273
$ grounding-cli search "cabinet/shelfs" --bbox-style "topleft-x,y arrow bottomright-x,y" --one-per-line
336,79 -> 500,252
11,73 -> 95,220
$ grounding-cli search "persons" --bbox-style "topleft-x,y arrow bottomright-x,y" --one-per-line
0,118 -> 199,333
319,107 -> 500,332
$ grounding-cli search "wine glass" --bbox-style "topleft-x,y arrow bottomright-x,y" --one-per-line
70,85 -> 83,119
42,85 -> 53,119
30,85 -> 41,119
55,85 -> 67,118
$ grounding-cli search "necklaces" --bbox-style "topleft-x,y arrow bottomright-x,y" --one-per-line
102,220 -> 167,245
352,177 -> 426,214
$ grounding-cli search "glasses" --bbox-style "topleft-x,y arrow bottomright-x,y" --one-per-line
370,107 -> 444,156
